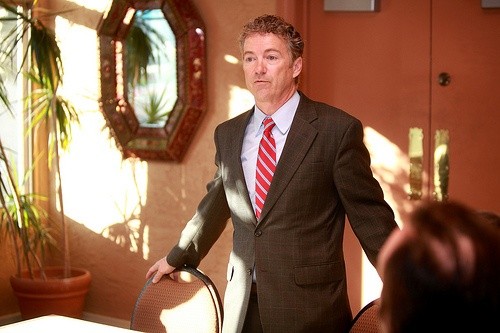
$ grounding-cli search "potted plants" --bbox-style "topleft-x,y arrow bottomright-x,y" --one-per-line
0,0 -> 91,320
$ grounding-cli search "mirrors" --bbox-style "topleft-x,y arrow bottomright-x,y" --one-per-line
95,0 -> 209,163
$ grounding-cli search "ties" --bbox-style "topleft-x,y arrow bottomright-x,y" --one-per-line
254,117 -> 277,222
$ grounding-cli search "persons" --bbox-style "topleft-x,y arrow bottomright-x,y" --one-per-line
376,196 -> 500,332
145,13 -> 398,332
